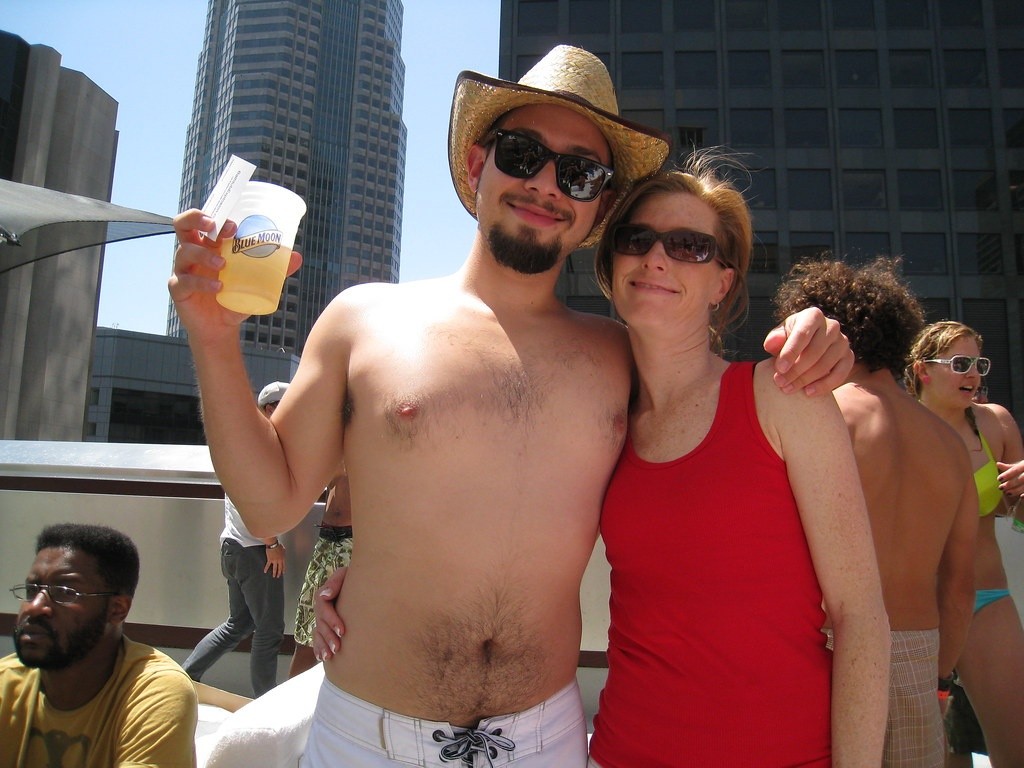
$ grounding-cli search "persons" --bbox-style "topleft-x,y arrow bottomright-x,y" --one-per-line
181,381 -> 293,689
172,44 -> 855,768
0,524 -> 199,768
313,168 -> 892,767
778,256 -> 979,767
901,319 -> 1024,768
287,470 -> 351,678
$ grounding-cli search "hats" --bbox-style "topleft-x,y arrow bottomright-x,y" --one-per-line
257,380 -> 290,406
448,43 -> 672,247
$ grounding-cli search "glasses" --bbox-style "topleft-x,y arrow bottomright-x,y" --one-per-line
489,128 -> 615,201
10,582 -> 116,604
922,355 -> 994,377
611,222 -> 739,269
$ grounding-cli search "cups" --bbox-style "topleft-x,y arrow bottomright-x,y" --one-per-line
215,180 -> 307,315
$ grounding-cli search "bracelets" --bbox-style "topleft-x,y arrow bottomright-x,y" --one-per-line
935,671 -> 955,698
265,540 -> 279,549
